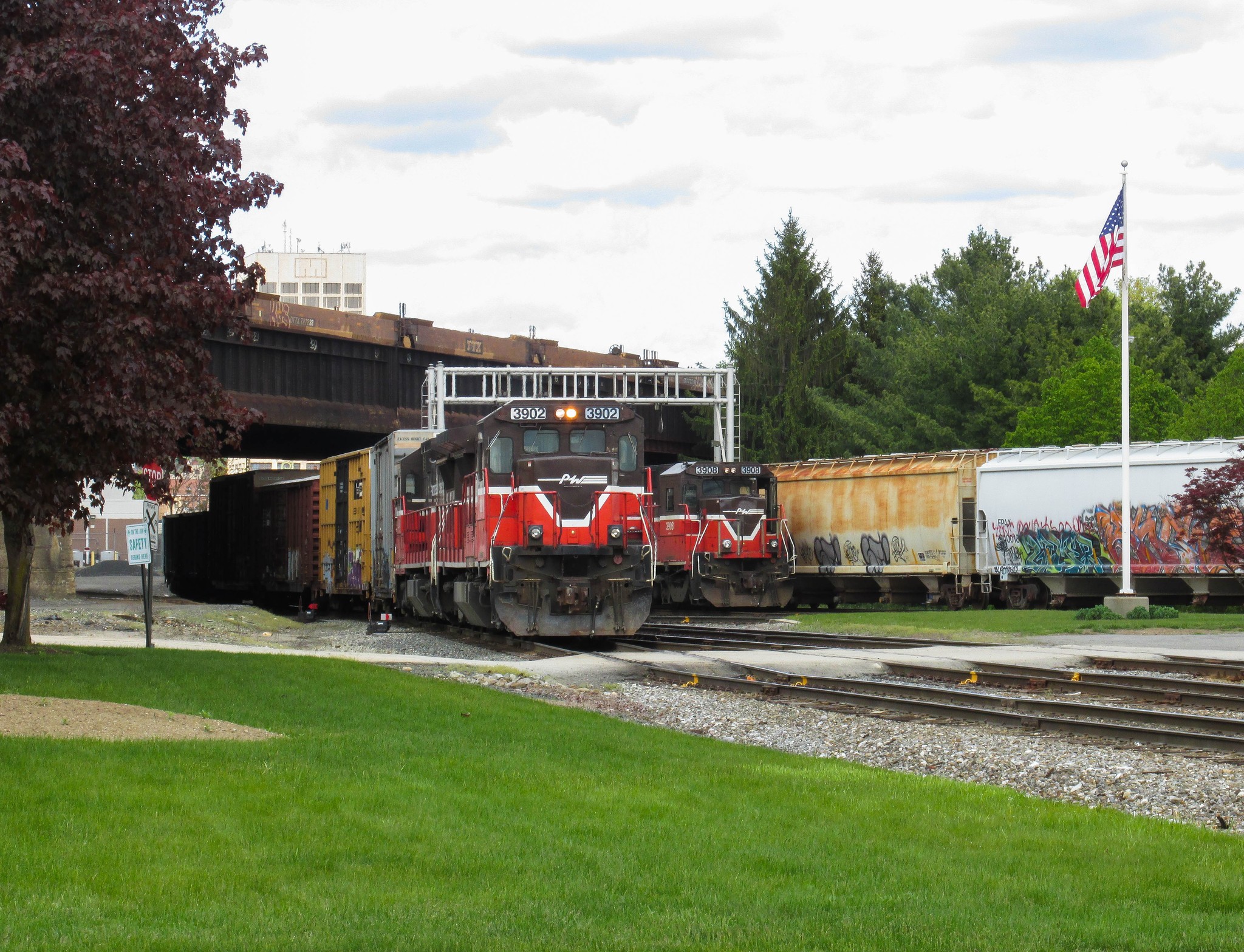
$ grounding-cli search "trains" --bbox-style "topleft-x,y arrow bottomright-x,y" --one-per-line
641,459 -> 799,610
735,433 -> 1244,615
161,400 -> 660,640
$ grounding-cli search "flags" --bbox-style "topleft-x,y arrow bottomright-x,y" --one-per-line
1076,182 -> 1124,308
